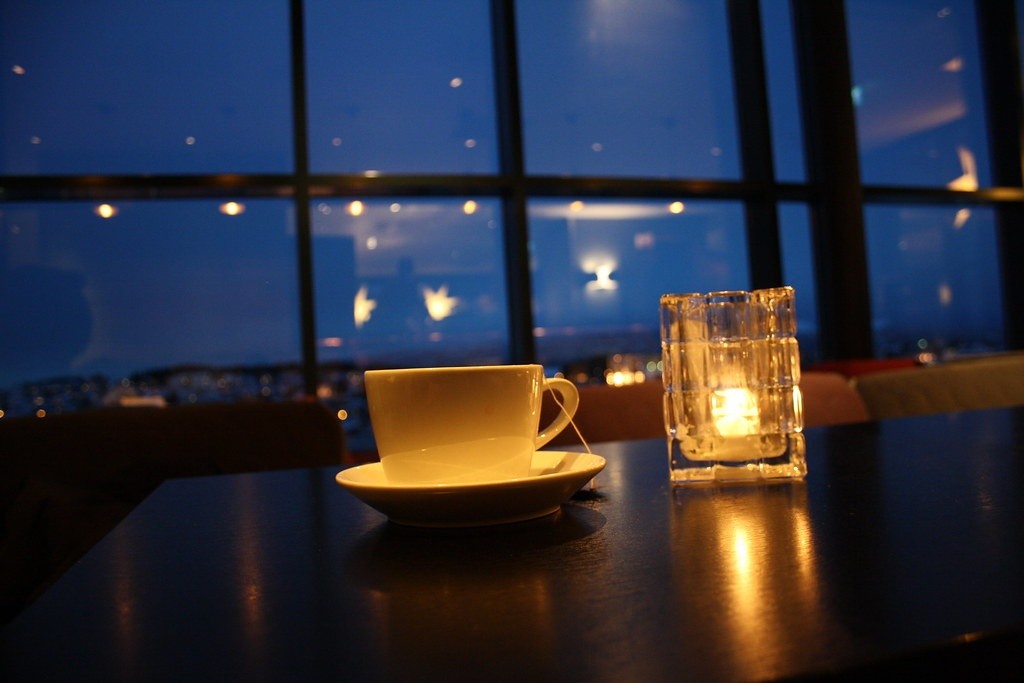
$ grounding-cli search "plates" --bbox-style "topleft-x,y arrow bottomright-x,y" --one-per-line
335,450 -> 606,528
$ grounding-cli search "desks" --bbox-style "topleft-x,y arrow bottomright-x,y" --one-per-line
0,402 -> 1024,683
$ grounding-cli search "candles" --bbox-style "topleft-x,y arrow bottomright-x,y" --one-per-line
657,287 -> 808,492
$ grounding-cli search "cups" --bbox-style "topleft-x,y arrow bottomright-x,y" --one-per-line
363,365 -> 580,481
659,286 -> 809,486
